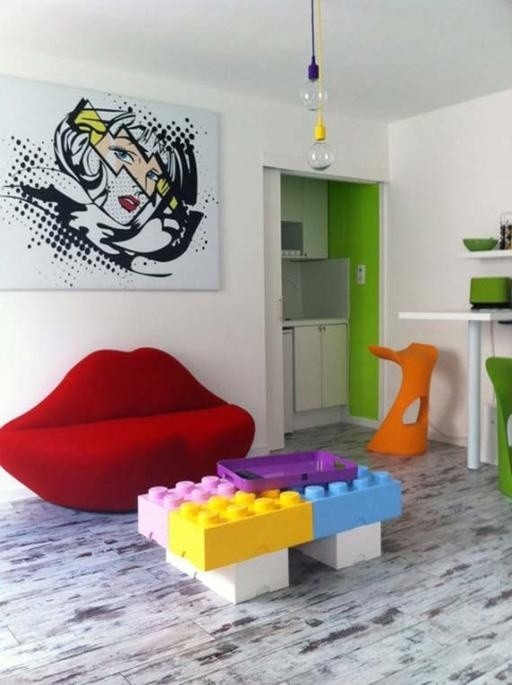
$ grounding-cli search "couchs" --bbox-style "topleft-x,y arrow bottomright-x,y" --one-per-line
0,347 -> 255,512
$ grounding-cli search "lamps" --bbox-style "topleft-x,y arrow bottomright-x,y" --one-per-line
300,1 -> 328,111
306,2 -> 334,171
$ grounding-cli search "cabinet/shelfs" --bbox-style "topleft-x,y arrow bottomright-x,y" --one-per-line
294,324 -> 349,430
280,173 -> 329,261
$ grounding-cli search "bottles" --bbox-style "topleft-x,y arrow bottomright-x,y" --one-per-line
499,211 -> 512,250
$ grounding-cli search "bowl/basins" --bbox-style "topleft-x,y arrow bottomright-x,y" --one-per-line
462,237 -> 499,251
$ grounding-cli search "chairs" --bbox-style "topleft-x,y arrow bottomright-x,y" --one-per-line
486,357 -> 512,501
365,342 -> 438,457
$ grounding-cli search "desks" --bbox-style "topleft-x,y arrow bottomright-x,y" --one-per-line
138,461 -> 402,605
398,309 -> 512,471
